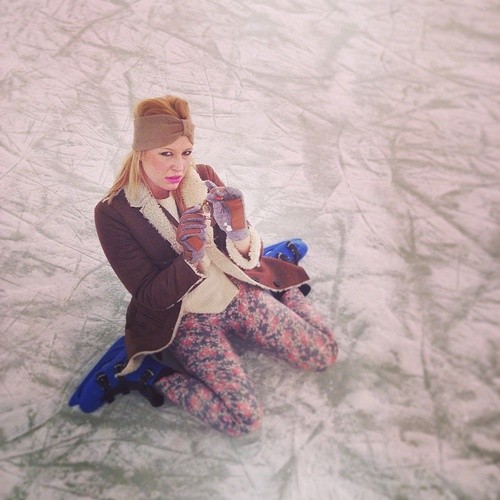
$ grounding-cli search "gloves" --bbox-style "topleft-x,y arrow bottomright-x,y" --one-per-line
176,204 -> 208,264
204,180 -> 250,241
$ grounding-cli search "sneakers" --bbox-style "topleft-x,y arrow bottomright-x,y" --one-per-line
261,236 -> 310,301
63,330 -> 176,413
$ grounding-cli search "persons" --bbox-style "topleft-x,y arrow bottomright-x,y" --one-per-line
69,96 -> 338,435
